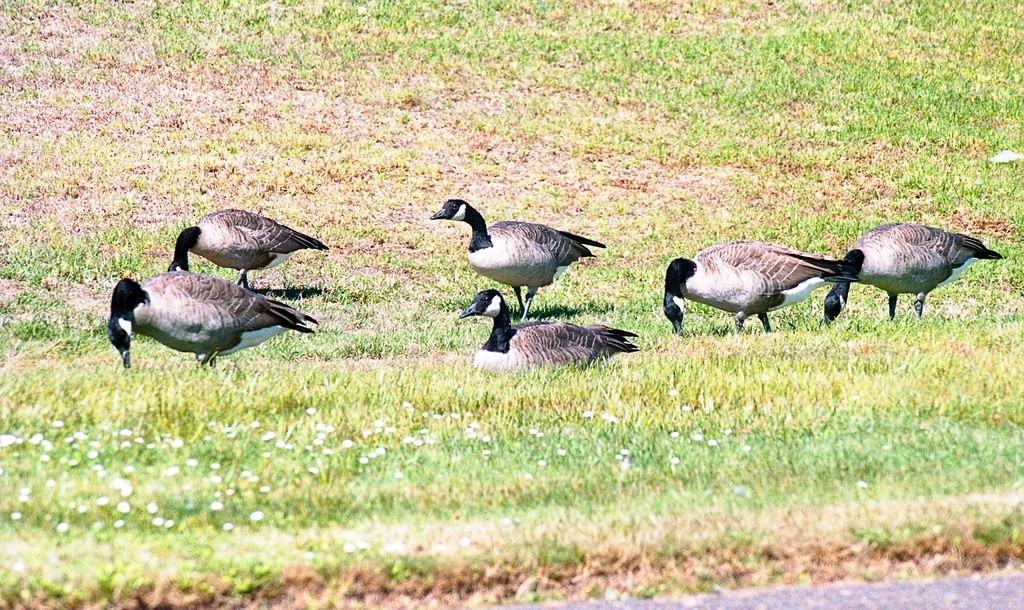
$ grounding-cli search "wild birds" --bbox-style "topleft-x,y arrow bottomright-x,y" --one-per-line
823,223 -> 1004,326
662,240 -> 861,337
167,209 -> 329,290
107,271 -> 320,370
429,198 -> 606,322
458,288 -> 641,372
